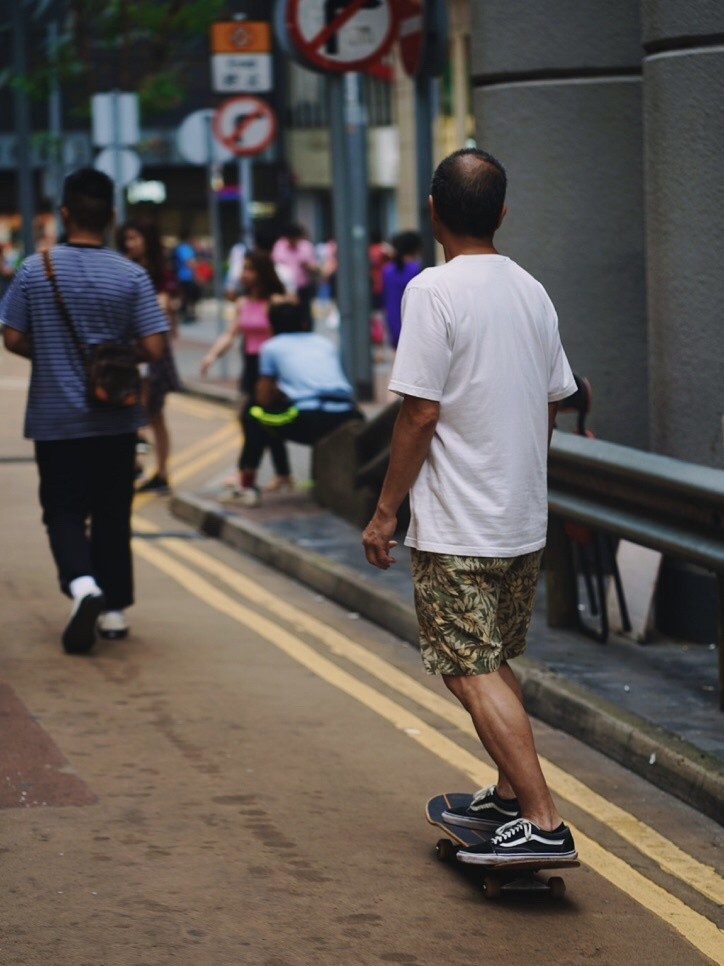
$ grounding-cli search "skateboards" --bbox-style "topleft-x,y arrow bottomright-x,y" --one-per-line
425,793 -> 580,902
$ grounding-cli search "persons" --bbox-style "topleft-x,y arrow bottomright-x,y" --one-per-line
217,298 -> 355,508
360,142 -> 584,869
197,254 -> 300,490
124,219 -> 204,490
0,202 -> 64,296
222,217 -> 394,343
384,231 -> 429,353
0,167 -> 171,652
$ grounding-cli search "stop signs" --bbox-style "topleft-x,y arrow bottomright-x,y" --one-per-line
396,1 -> 426,74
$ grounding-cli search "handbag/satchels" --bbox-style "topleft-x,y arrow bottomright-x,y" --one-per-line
88,341 -> 141,413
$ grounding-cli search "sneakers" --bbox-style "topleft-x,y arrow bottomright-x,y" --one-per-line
442,785 -> 521,832
456,818 -> 578,864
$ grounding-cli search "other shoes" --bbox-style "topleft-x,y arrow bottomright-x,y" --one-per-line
137,475 -> 170,493
61,577 -> 104,655
96,613 -> 129,640
217,485 -> 263,508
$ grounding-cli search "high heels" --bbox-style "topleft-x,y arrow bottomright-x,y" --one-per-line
266,477 -> 293,494
